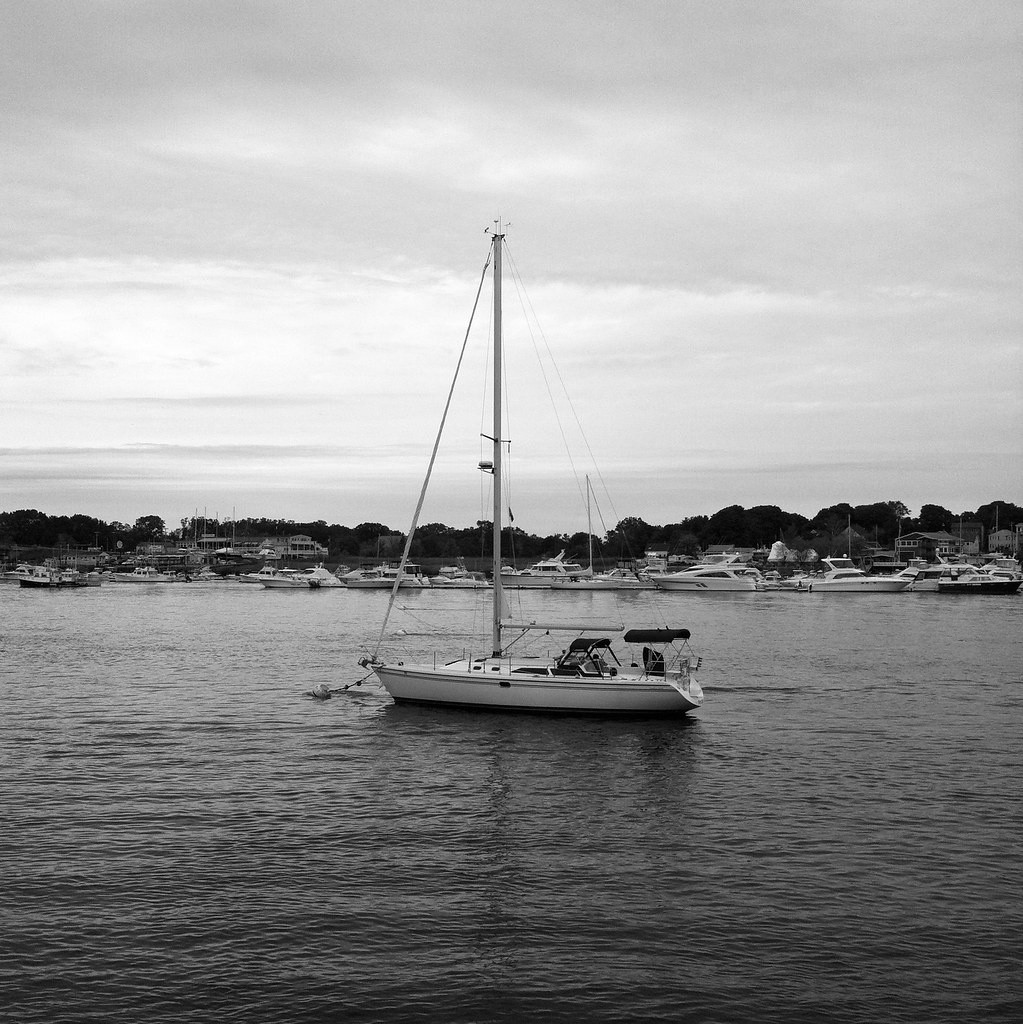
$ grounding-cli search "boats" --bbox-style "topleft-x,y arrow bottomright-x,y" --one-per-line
0,471 -> 1022,593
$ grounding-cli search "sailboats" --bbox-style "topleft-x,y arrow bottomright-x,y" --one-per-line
362,228 -> 714,716
